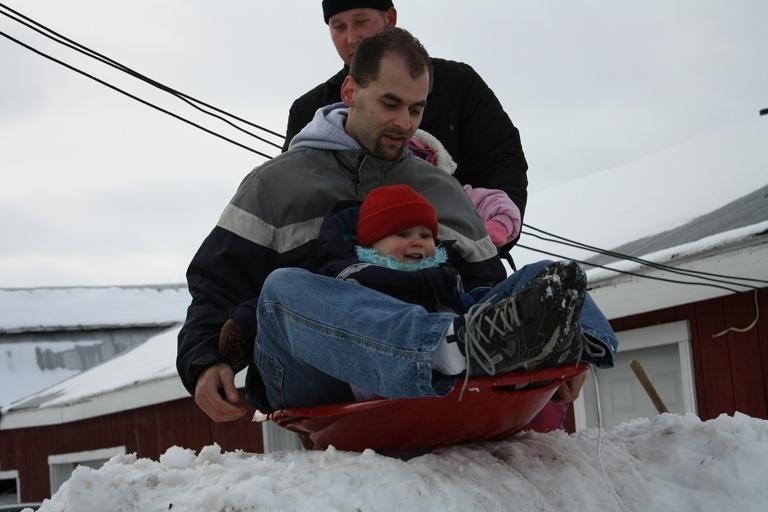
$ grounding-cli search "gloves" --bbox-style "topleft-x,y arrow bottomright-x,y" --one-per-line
485,220 -> 507,247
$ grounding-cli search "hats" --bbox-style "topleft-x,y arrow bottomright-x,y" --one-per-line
356,184 -> 438,249
322,0 -> 394,25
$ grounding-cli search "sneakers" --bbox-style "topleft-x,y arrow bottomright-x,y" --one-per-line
440,278 -> 569,384
525,258 -> 587,369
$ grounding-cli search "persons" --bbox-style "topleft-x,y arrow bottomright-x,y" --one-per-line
175,28 -> 620,423
407,125 -> 522,247
217,183 -> 469,406
279,0 -> 529,259
